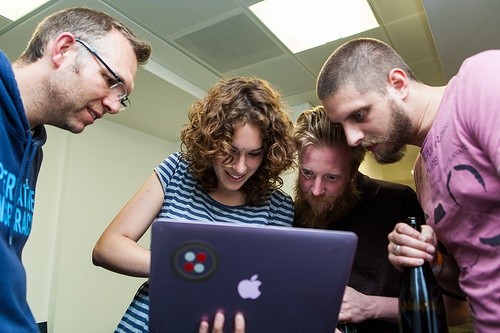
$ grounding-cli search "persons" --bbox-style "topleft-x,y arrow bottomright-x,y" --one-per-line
92,76 -> 295,333
315,37 -> 500,332
0,5 -> 153,333
294,104 -> 469,332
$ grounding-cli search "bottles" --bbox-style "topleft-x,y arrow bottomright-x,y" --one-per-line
398,217 -> 449,333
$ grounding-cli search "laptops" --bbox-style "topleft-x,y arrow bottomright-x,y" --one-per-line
149,218 -> 357,333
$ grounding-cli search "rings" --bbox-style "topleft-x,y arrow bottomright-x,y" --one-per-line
393,244 -> 401,255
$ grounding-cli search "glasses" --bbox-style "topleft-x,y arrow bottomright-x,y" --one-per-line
75,38 -> 131,112
